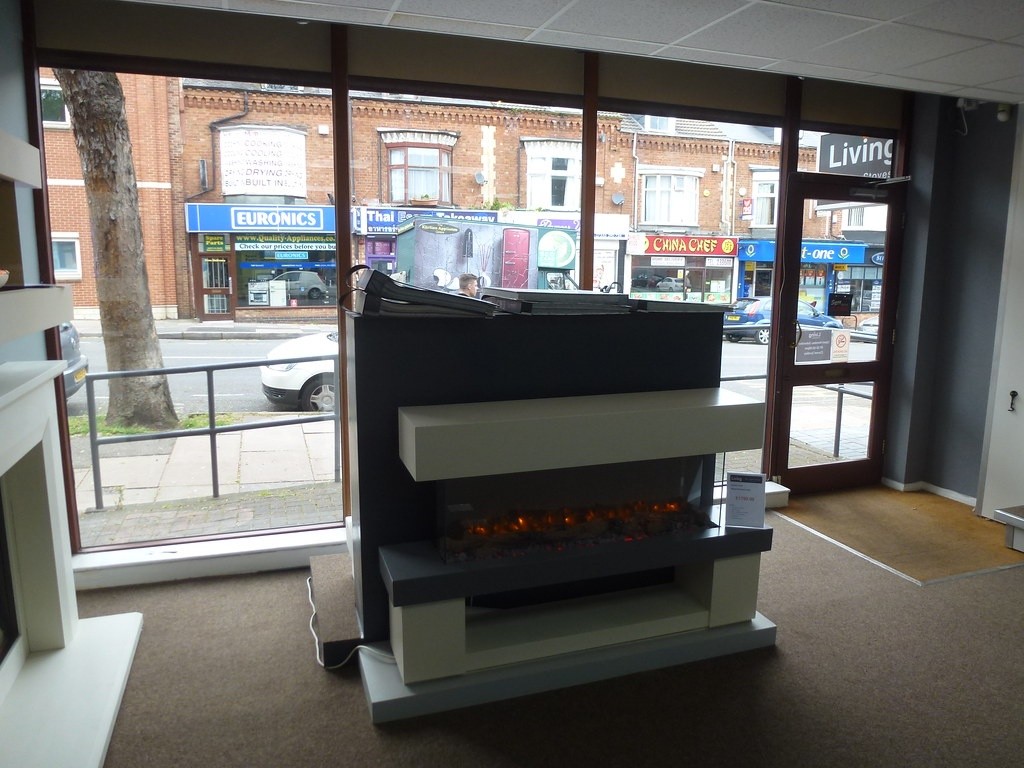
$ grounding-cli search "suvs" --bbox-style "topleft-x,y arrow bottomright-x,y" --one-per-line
722,295 -> 842,347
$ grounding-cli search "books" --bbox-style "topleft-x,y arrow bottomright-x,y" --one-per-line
337,290 -> 488,319
629,297 -> 734,314
482,286 -> 629,305
481,294 -> 632,316
345,264 -> 500,317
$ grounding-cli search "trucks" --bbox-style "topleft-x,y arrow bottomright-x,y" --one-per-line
395,213 -> 578,303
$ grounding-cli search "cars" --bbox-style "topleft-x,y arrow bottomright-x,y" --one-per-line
58,321 -> 88,399
632,271 -> 664,288
260,328 -> 335,414
656,275 -> 691,291
268,270 -> 328,301
859,315 -> 880,335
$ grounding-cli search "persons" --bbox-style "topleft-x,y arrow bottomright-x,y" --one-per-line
457,272 -> 479,299
433,275 -> 444,289
